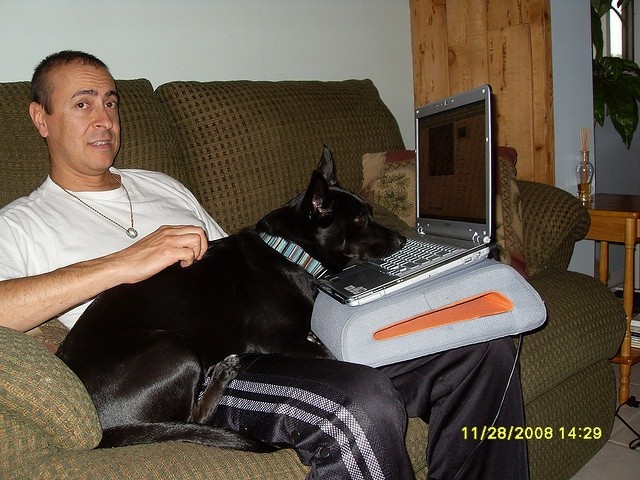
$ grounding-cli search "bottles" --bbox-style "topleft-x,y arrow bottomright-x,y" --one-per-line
575,150 -> 594,202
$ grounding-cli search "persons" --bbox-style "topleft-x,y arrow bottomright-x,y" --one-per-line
0,50 -> 532,480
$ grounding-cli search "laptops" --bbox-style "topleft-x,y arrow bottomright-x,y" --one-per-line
317,85 -> 493,307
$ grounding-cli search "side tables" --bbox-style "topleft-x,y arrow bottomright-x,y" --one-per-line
576,192 -> 640,406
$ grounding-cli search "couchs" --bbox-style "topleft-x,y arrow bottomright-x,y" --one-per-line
0,77 -> 627,480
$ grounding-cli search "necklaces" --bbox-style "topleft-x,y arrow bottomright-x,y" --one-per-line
53,175 -> 139,239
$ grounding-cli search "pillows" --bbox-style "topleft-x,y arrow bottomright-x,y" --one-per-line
359,145 -> 530,284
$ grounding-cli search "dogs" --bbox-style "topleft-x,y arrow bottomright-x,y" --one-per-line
53,143 -> 407,453
